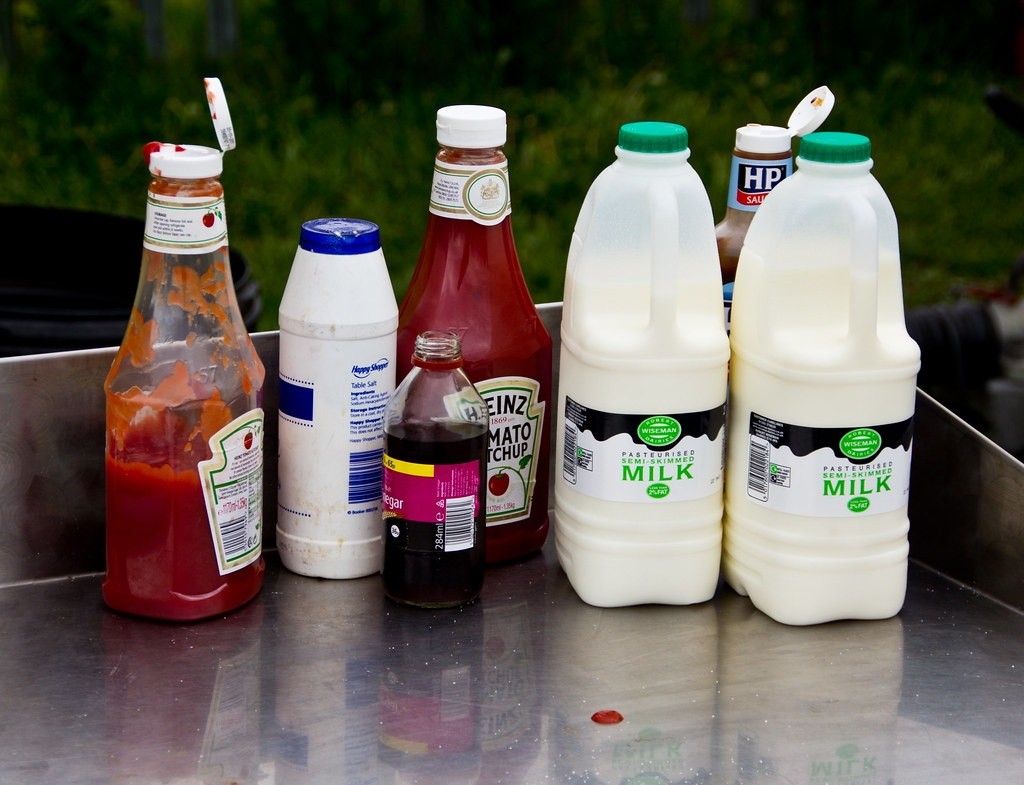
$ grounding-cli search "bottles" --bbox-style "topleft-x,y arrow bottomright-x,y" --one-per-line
713,87 -> 835,383
397,106 -> 551,564
557,122 -> 732,609
725,132 -> 920,626
104,78 -> 265,619
384,331 -> 485,609
275,218 -> 399,579
